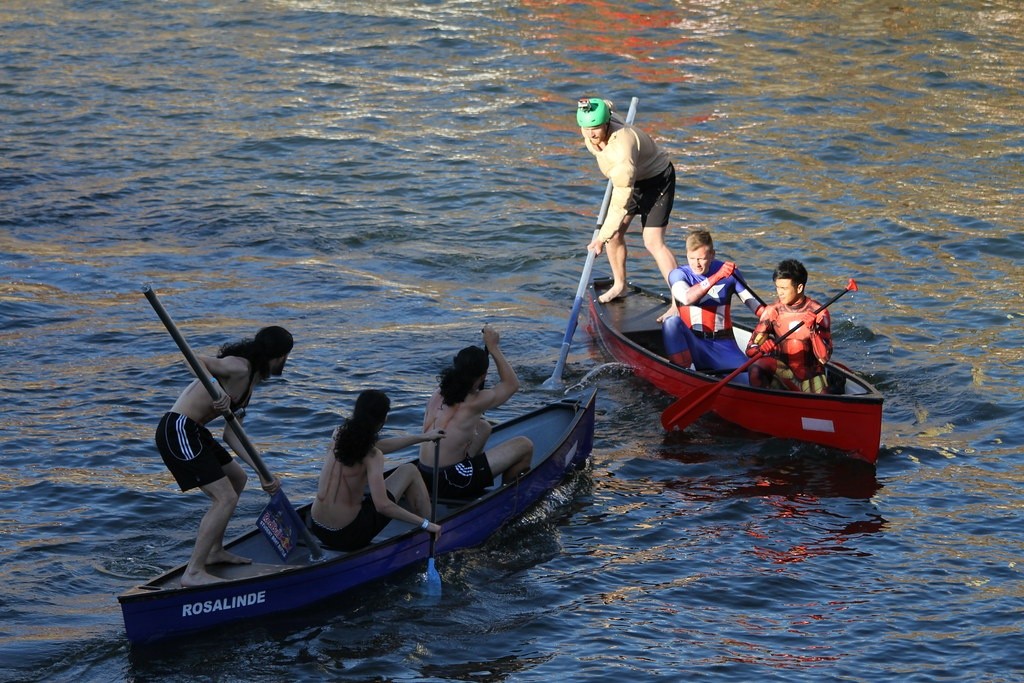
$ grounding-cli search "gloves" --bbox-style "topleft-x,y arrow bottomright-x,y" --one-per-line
755,305 -> 767,317
802,310 -> 816,330
688,261 -> 738,304
759,338 -> 777,353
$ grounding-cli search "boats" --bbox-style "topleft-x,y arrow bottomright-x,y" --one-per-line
587,272 -> 884,468
116,385 -> 602,668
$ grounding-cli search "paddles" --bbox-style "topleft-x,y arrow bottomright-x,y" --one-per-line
731,272 -> 854,373
422,437 -> 441,596
660,277 -> 860,432
477,328 -> 488,390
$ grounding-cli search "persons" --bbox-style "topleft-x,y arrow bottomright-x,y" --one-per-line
155,325 -> 294,585
416,324 -> 534,500
662,231 -> 767,385
577,98 -> 681,322
308,389 -> 447,552
745,259 -> 833,395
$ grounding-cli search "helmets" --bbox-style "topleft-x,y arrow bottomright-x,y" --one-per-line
576,97 -> 610,127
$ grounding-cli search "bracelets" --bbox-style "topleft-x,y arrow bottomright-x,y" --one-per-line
209,377 -> 218,383
422,517 -> 429,529
